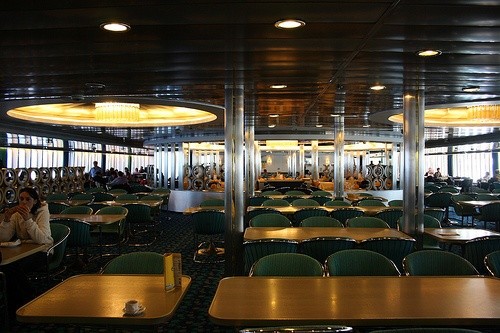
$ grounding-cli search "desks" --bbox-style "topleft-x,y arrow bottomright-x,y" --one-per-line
246,206 -> 417,215
423,228 -> 500,272
16,274 -> 192,333
183,206 -> 226,256
266,195 -> 388,202
54,200 -> 90,206
0,243 -> 45,321
208,276 -> 500,333
50,214 -> 125,264
458,200 -> 500,229
88,200 -> 164,240
243,227 -> 417,255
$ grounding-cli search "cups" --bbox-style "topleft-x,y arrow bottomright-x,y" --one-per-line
126,300 -> 138,314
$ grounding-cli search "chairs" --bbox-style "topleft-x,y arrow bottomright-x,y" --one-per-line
47,176 -> 500,289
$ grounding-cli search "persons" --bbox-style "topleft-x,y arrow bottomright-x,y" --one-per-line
85,161 -> 145,185
424,168 -> 442,177
0,187 -> 54,284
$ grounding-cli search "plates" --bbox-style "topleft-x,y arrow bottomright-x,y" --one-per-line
121,305 -> 146,314
